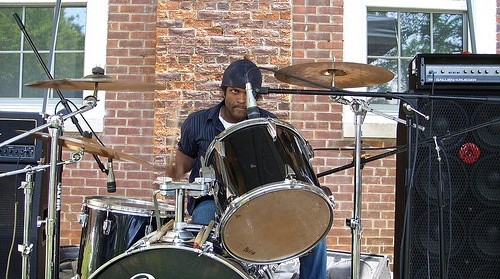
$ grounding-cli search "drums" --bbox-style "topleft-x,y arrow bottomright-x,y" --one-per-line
89,223 -> 301,279
202,116 -> 334,265
74,195 -> 183,279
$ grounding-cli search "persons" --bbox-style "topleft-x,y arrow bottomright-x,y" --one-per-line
165,60 -> 327,279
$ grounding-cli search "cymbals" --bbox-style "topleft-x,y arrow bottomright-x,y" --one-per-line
274,61 -> 394,88
16,129 -> 152,168
24,73 -> 166,92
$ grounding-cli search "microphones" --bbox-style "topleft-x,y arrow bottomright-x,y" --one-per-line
245,73 -> 260,120
106,158 -> 116,193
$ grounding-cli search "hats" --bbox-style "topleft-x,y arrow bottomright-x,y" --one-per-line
222,60 -> 262,88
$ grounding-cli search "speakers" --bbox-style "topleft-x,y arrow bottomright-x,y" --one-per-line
0,164 -> 55,279
393,89 -> 500,279
